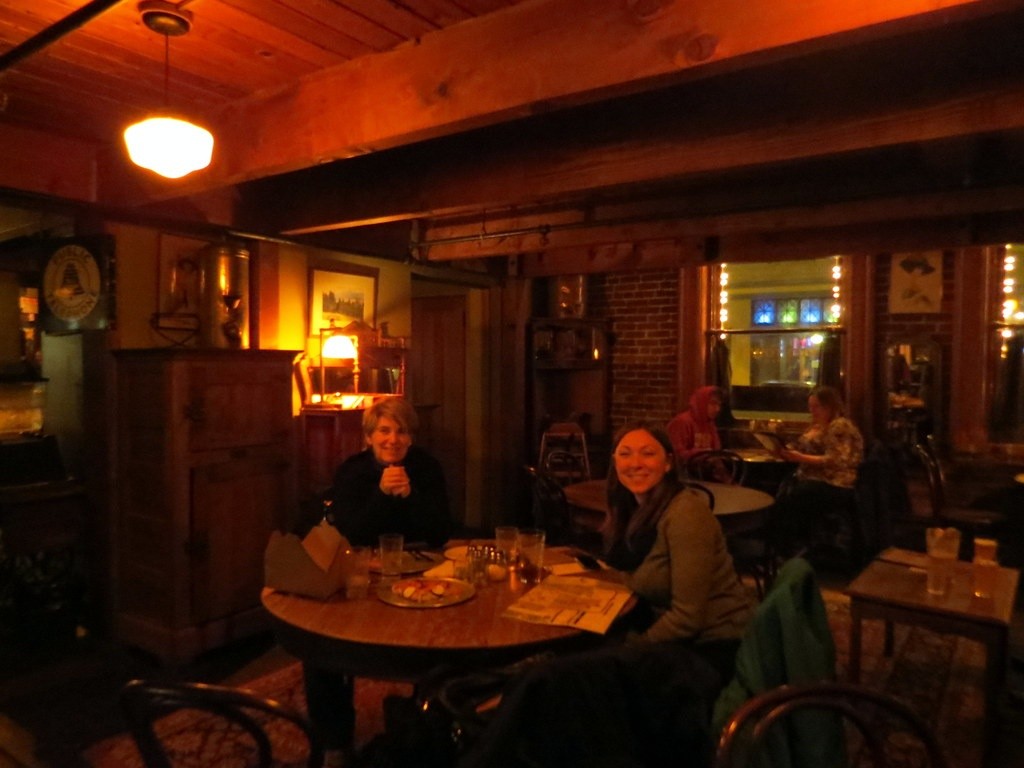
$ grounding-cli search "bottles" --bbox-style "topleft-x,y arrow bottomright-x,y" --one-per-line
465,543 -> 504,580
972,539 -> 997,597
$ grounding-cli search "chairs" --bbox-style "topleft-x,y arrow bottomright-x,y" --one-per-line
118,427 -> 1024,768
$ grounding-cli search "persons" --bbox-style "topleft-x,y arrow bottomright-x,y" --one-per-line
663,385 -> 728,482
525,420 -> 749,744
767,385 -> 865,559
322,396 -> 450,546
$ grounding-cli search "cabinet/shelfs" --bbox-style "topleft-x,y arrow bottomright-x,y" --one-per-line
526,317 -> 613,477
109,344 -> 304,664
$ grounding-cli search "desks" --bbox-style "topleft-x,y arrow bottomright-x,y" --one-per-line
300,407 -> 365,487
560,478 -> 777,535
259,536 -> 642,674
723,446 -> 800,472
840,545 -> 1021,704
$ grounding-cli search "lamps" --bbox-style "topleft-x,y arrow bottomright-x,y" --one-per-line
305,328 -> 357,409
120,0 -> 214,178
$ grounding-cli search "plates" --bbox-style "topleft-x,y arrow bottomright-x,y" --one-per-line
368,552 -> 444,574
374,577 -> 475,607
444,545 -> 469,561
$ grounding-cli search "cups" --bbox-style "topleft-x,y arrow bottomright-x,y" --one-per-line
495,525 -> 518,568
345,546 -> 372,599
926,528 -> 959,594
515,528 -> 545,584
364,396 -> 374,407
380,532 -> 403,582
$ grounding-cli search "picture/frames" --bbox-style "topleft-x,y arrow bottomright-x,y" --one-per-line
307,260 -> 379,336
156,232 -> 212,332
